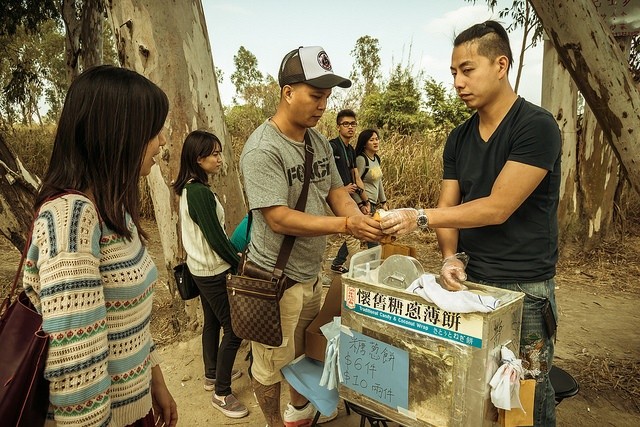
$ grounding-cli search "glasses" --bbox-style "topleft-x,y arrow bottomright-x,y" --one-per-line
339,121 -> 357,126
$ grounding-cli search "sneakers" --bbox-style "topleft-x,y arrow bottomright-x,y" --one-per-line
212,392 -> 249,418
204,370 -> 241,391
320,271 -> 332,285
330,264 -> 349,274
283,402 -> 338,427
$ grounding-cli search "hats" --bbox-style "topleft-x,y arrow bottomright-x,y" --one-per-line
279,45 -> 352,89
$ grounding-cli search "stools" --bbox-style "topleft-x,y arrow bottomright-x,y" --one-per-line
549,366 -> 580,407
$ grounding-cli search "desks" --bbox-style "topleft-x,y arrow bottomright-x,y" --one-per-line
280,352 -> 530,427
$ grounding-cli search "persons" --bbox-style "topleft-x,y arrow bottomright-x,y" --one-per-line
378,19 -> 564,427
325,108 -> 391,274
239,44 -> 383,427
170,130 -> 249,418
24,64 -> 178,427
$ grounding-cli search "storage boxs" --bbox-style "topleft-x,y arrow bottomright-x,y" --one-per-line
338,258 -> 526,427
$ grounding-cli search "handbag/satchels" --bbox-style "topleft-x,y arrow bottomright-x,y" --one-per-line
225,273 -> 283,347
173,264 -> 200,301
244,261 -> 286,304
0,188 -> 104,427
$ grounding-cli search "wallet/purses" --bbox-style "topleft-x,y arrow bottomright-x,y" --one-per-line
542,301 -> 557,344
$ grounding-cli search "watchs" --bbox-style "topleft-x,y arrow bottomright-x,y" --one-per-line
416,208 -> 428,229
